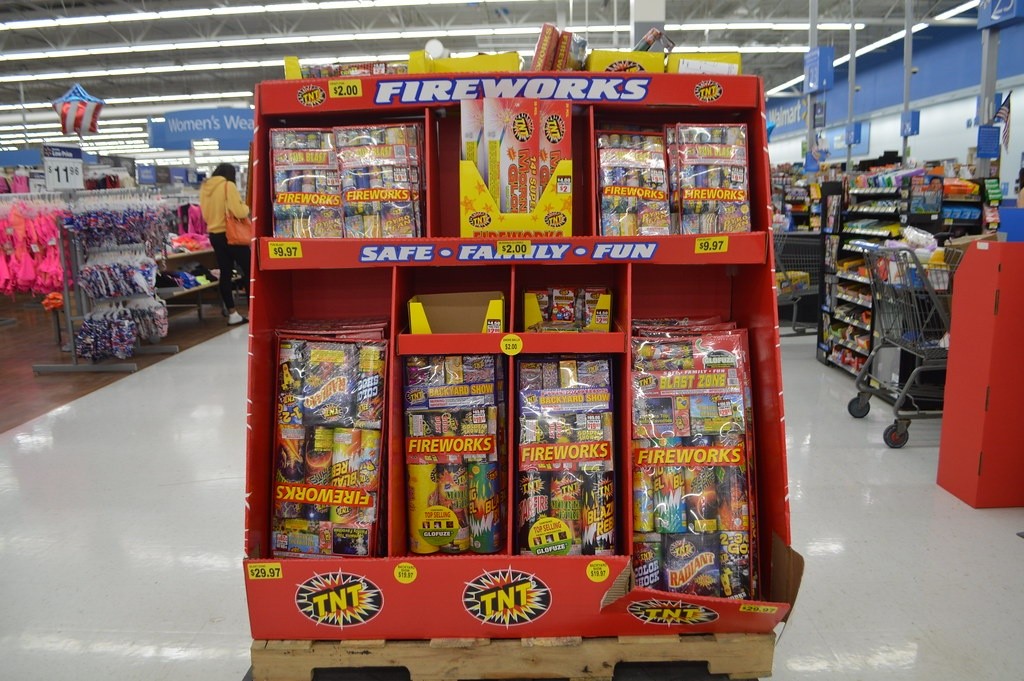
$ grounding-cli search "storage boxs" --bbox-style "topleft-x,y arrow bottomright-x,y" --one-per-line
846,284 -> 858,297
858,265 -> 872,277
856,336 -> 870,349
241,22 -> 804,635
775,270 -> 809,294
836,282 -> 846,293
857,288 -> 872,303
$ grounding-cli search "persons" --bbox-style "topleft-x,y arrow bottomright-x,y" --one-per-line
199,164 -> 250,324
917,178 -> 942,211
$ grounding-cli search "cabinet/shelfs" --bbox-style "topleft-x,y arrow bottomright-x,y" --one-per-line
780,181 -> 988,411
155,249 -> 243,319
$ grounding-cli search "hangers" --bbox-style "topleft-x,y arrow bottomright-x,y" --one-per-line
70,186 -> 164,212
81,244 -> 153,265
86,302 -> 129,321
0,191 -> 64,206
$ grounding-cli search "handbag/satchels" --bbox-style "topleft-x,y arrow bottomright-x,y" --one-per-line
225,215 -> 252,246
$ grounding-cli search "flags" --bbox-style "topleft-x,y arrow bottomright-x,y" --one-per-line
996,94 -> 1011,152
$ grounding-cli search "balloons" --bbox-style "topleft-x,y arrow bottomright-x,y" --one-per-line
53,83 -> 105,136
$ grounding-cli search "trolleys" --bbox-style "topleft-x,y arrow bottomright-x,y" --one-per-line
772,232 -> 823,336
847,240 -> 950,449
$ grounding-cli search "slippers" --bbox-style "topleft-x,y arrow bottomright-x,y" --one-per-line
227,314 -> 249,326
